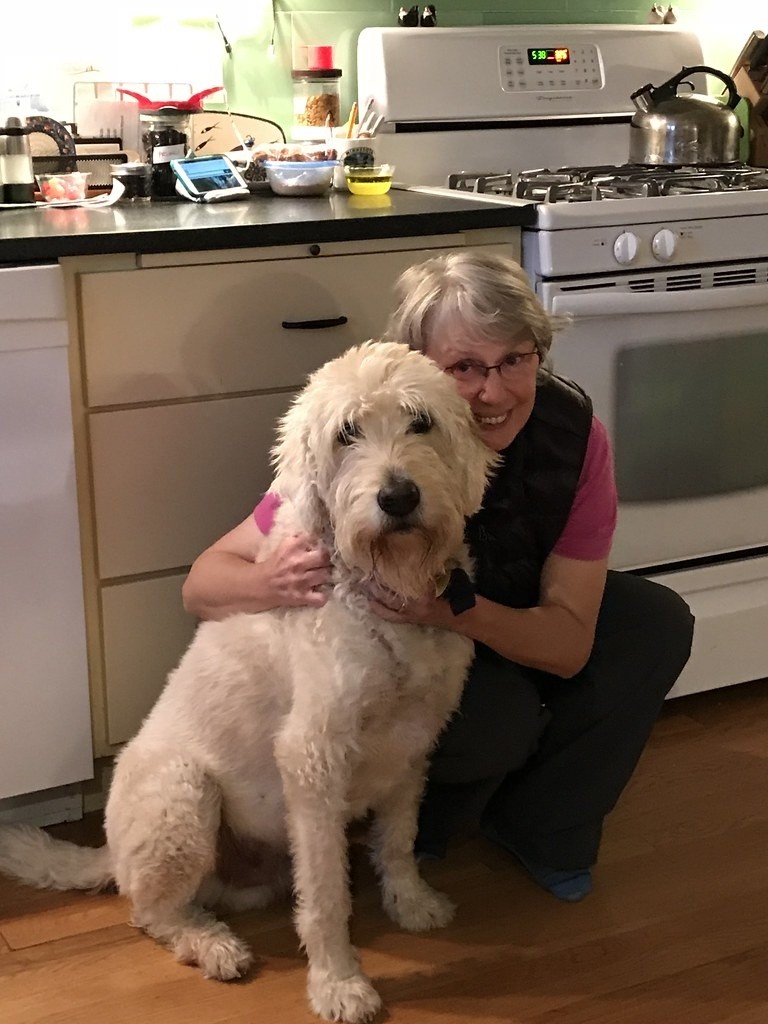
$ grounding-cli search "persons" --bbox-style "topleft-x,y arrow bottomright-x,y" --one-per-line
180,251 -> 698,898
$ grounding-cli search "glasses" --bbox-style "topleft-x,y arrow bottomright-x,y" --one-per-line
443,352 -> 542,381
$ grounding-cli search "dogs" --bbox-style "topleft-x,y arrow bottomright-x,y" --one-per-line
0,337 -> 505,1023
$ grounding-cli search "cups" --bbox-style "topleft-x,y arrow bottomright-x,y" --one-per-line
110,164 -> 151,203
327,137 -> 375,190
345,163 -> 396,195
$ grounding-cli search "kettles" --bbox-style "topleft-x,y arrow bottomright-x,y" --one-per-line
628,65 -> 744,167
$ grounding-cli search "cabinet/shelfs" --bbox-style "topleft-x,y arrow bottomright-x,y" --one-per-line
61,226 -> 524,763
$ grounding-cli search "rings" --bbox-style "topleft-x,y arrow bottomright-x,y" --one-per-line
399,599 -> 406,612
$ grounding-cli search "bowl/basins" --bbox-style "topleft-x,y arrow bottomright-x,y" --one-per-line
260,160 -> 339,195
36,172 -> 90,203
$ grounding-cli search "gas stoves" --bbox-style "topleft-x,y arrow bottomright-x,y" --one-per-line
355,23 -> 768,276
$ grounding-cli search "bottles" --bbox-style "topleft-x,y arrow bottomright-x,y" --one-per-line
289,68 -> 342,139
0,116 -> 34,204
139,110 -> 192,200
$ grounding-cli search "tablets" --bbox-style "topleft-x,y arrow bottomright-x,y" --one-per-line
170,154 -> 248,196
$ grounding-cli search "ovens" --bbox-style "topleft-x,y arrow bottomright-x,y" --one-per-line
526,263 -> 768,701
